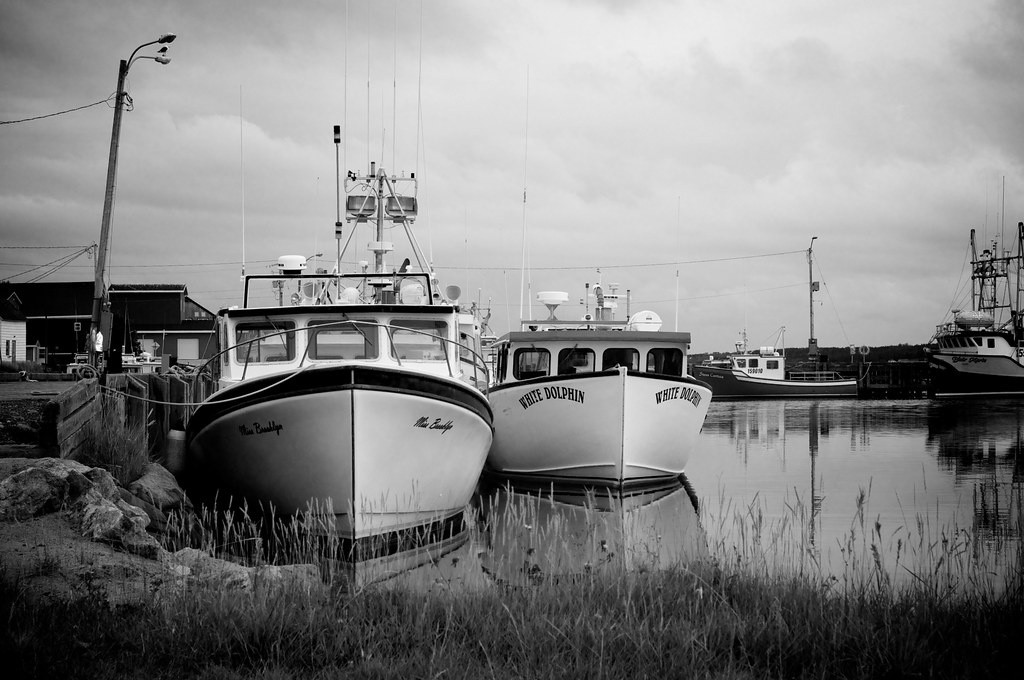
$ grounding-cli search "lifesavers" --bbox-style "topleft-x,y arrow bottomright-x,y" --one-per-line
859,344 -> 870,355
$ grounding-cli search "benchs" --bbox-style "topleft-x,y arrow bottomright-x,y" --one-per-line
66,364 -> 89,374
123,362 -> 143,372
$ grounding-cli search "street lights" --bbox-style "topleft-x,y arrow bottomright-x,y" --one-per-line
85,32 -> 179,375
809,236 -> 819,369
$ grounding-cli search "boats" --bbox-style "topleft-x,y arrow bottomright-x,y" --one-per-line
917,175 -> 1024,400
482,470 -> 712,611
214,507 -> 472,596
688,311 -> 859,401
183,0 -> 497,520
478,63 -> 715,491
